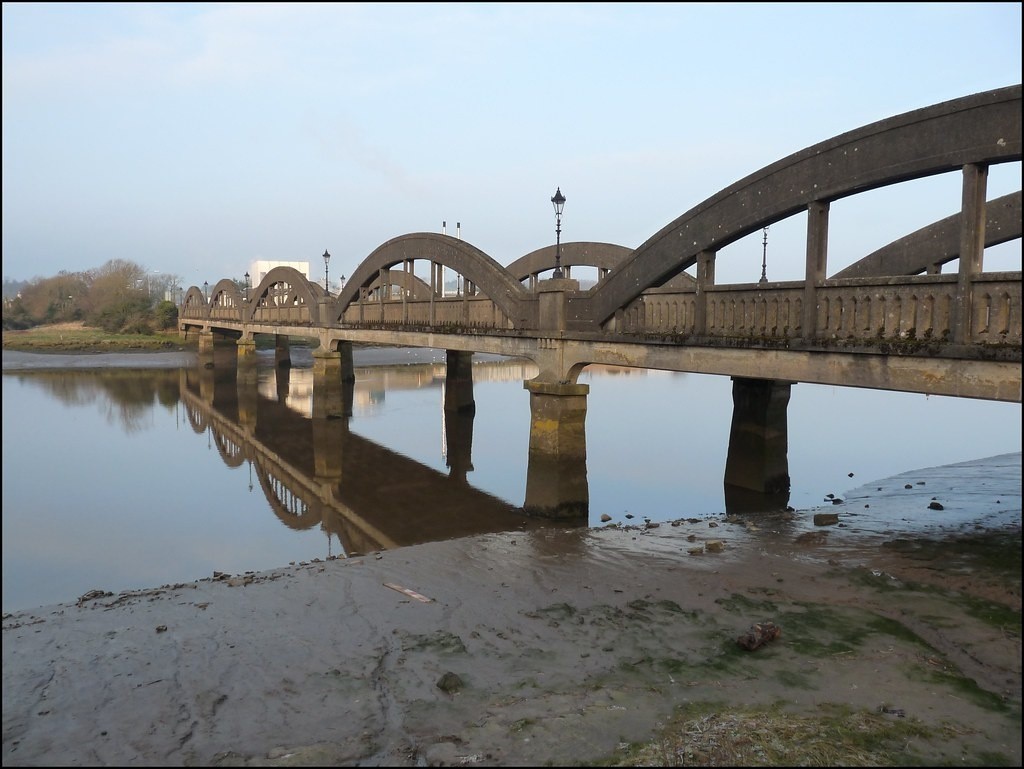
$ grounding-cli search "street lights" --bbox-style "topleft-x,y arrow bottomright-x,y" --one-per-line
204,280 -> 208,304
339,274 -> 346,294
179,286 -> 183,307
760,224 -> 770,284
323,248 -> 330,293
244,270 -> 250,302
550,186 -> 570,279
